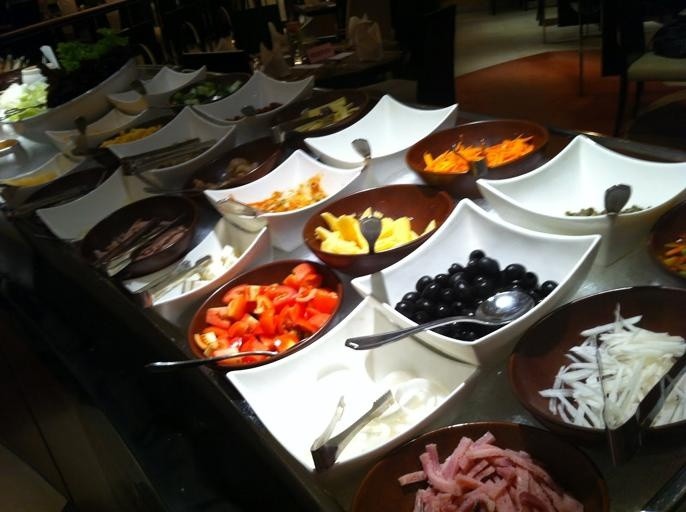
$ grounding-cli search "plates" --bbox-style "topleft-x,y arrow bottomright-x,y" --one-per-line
104,107 -> 238,188
44,108 -> 149,151
305,93 -> 461,187
124,216 -> 275,339
107,65 -> 209,113
35,164 -> 203,277
192,70 -> 315,126
228,295 -> 482,472
476,134 -> 686,270
3,151 -> 86,193
349,197 -> 603,373
208,151 -> 364,221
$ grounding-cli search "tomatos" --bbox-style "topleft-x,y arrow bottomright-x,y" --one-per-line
193,262 -> 337,364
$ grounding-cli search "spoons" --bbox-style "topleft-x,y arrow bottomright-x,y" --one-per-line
344,290 -> 533,351
148,347 -> 280,368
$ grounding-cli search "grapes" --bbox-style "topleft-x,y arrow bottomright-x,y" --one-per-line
395,249 -> 557,341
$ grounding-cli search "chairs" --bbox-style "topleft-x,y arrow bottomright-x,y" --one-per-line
535,2 -> 686,146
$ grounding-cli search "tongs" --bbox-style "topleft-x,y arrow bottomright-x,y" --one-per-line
309,390 -> 392,481
591,332 -> 686,461
132,254 -> 210,310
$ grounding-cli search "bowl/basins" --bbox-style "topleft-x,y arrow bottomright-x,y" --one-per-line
350,419 -> 615,512
649,199 -> 686,283
302,184 -> 458,279
188,258 -> 347,372
406,119 -> 552,200
507,283 -> 686,447
